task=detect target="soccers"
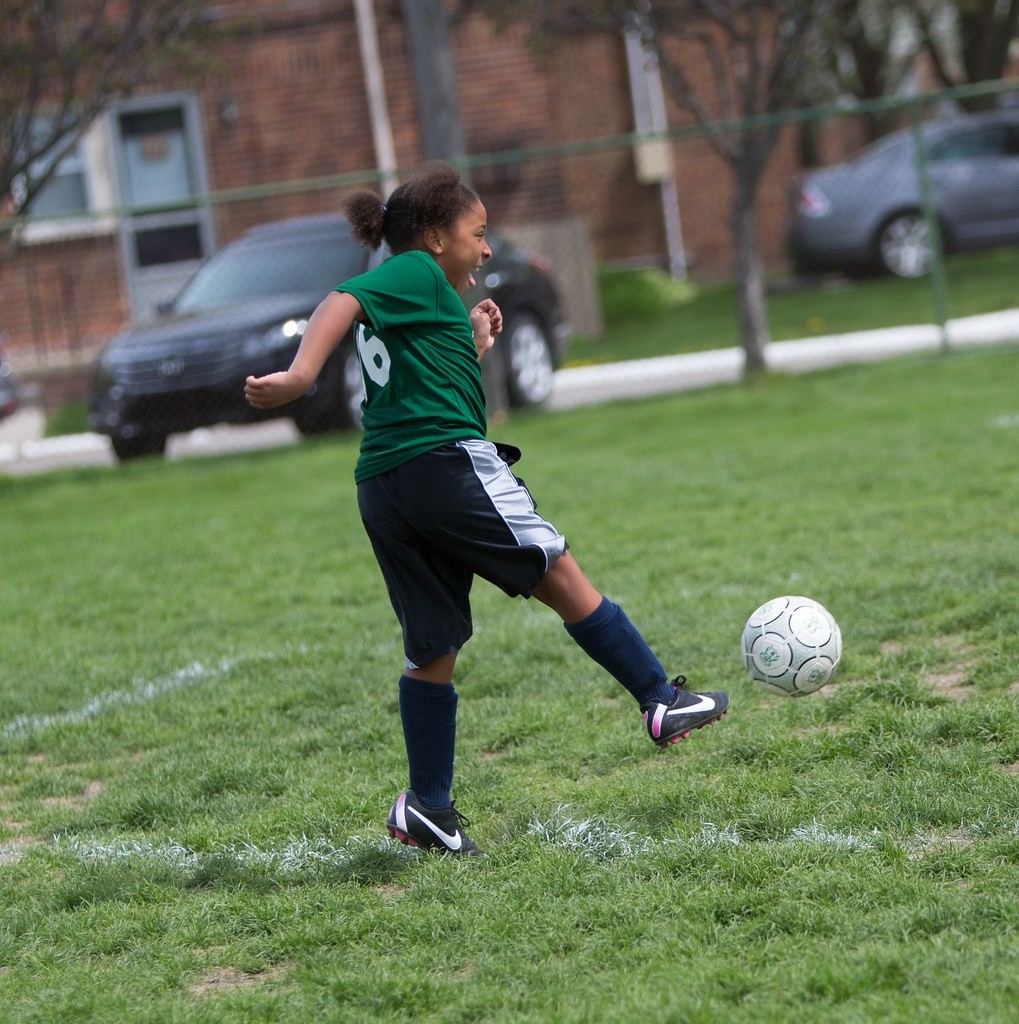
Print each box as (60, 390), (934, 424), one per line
(740, 595), (844, 698)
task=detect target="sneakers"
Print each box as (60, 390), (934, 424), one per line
(388, 788), (483, 858)
(646, 675), (730, 748)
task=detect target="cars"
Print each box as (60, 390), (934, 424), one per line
(87, 208), (571, 466)
(781, 110), (1017, 282)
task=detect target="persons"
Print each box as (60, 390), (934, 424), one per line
(243, 168), (729, 855)
